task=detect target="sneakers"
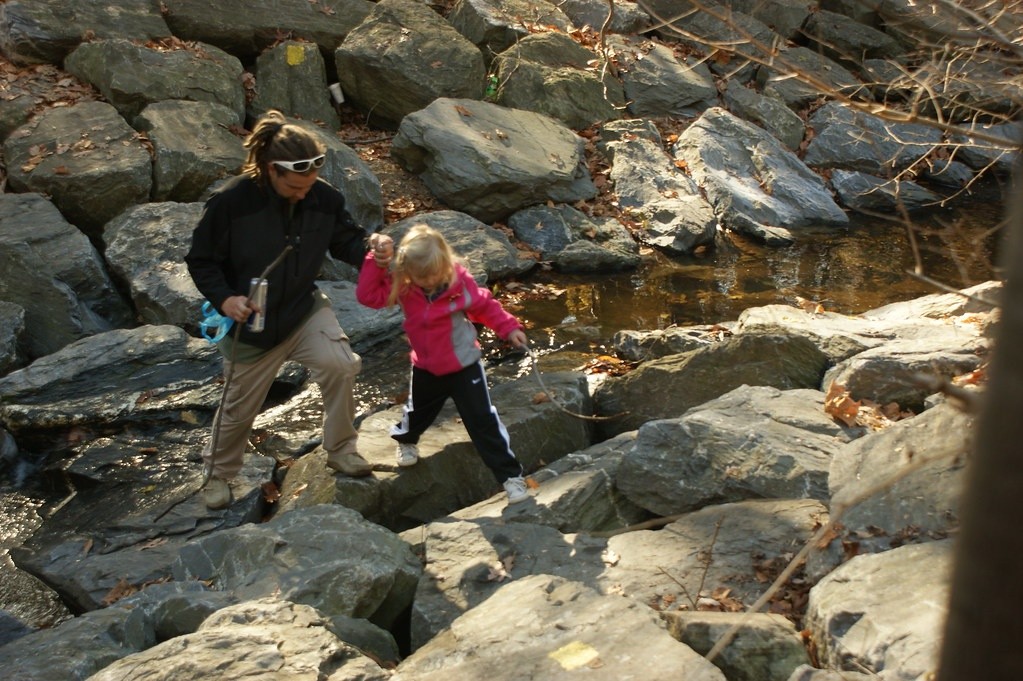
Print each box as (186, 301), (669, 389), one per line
(503, 477), (528, 504)
(396, 442), (419, 466)
(202, 466), (230, 508)
(327, 452), (373, 476)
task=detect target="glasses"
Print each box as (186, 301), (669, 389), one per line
(268, 154), (326, 172)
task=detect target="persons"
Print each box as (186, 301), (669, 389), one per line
(183, 109), (395, 510)
(356, 223), (530, 505)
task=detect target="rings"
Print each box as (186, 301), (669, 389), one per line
(388, 256), (392, 262)
